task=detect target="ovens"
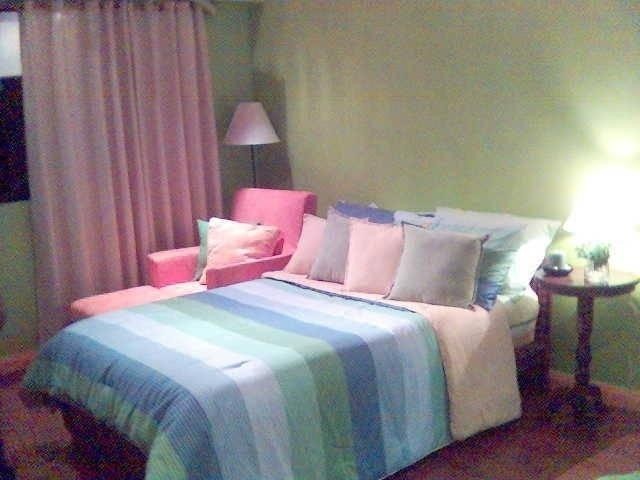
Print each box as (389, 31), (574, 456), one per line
(70, 189), (317, 320)
(18, 281), (550, 480)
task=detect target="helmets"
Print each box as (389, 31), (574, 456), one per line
(542, 262), (574, 275)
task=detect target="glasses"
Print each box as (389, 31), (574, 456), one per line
(224, 103), (281, 189)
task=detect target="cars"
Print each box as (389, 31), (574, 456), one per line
(550, 254), (563, 266)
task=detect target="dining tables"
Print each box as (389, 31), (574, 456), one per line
(576, 243), (611, 286)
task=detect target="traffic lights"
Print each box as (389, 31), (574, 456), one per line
(200, 217), (281, 284)
(192, 219), (208, 281)
(286, 202), (562, 311)
